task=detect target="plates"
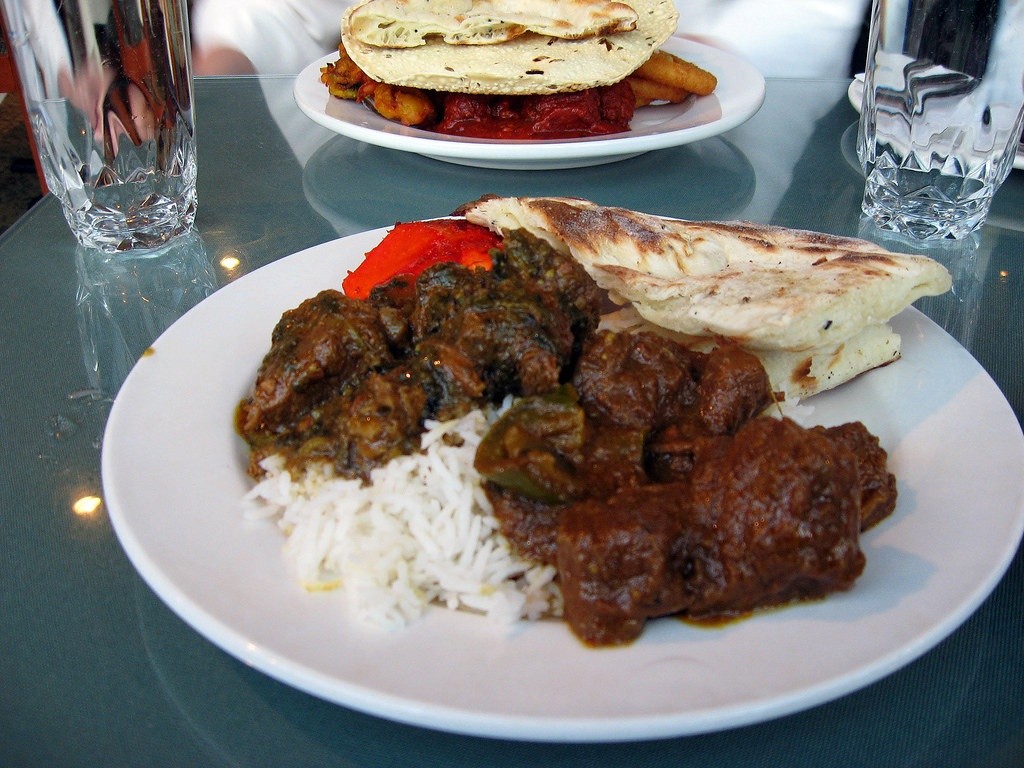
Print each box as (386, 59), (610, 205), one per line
(301, 135), (756, 235)
(99, 221), (1024, 743)
(848, 79), (1024, 170)
(293, 38), (767, 170)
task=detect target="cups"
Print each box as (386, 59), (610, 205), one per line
(0, 0), (198, 251)
(77, 238), (217, 392)
(848, 212), (986, 350)
(857, 0), (1024, 244)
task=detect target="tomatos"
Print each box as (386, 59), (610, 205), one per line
(342, 220), (504, 296)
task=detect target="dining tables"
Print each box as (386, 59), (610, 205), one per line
(0, 77), (1024, 768)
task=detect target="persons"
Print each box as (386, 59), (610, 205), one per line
(190, 0), (870, 79)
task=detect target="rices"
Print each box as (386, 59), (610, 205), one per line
(245, 396), (564, 628)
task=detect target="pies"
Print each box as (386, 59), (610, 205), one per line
(340, 0), (679, 96)
(465, 196), (952, 399)
